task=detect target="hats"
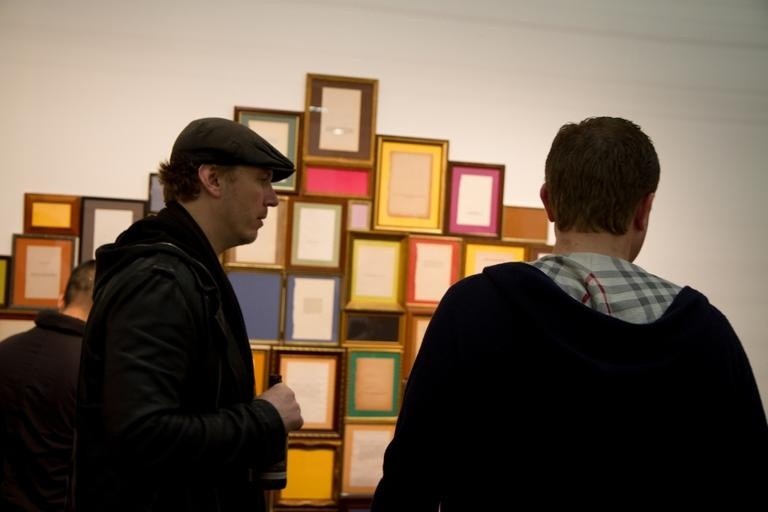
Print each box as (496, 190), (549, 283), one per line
(171, 118), (295, 183)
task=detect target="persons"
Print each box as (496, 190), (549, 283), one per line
(365, 114), (768, 511)
(1, 260), (100, 510)
(70, 118), (303, 511)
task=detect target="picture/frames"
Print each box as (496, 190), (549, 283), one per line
(2, 172), (166, 338)
(408, 233), (556, 310)
(340, 227), (411, 314)
(246, 345), (341, 512)
(444, 159), (506, 239)
(220, 198), (340, 347)
(301, 73), (379, 171)
(340, 311), (433, 425)
(370, 134), (449, 234)
(232, 105), (305, 196)
(286, 195), (349, 277)
